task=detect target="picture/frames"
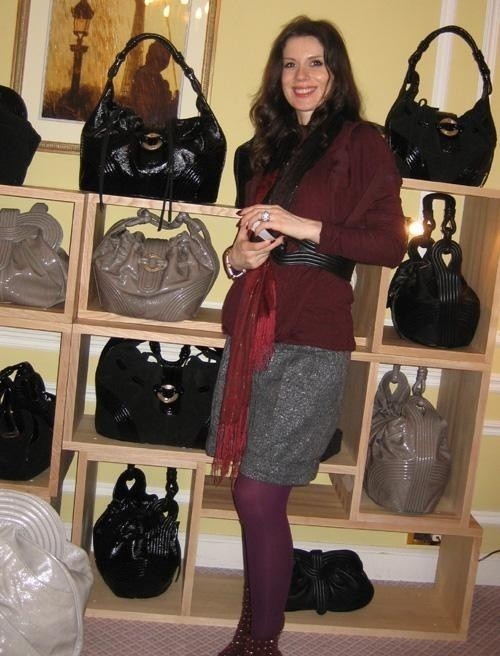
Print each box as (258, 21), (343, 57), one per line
(11, 0), (221, 157)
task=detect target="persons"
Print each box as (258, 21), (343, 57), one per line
(131, 40), (171, 126)
(205, 17), (408, 656)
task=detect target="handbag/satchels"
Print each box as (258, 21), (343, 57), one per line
(95, 336), (223, 449)
(92, 465), (181, 598)
(0, 361), (56, 481)
(285, 548), (374, 613)
(0, 85), (41, 185)
(0, 203), (69, 308)
(79, 33), (226, 204)
(91, 208), (220, 322)
(362, 364), (452, 514)
(385, 24), (496, 189)
(0, 489), (95, 656)
(386, 192), (481, 350)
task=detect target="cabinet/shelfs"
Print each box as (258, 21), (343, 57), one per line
(0, 184), (85, 323)
(190, 516), (483, 642)
(62, 324), (228, 463)
(371, 179), (499, 364)
(0, 317), (73, 497)
(78, 193), (243, 333)
(348, 355), (490, 527)
(350, 262), (382, 354)
(71, 451), (200, 623)
(202, 459), (355, 520)
(318, 352), (377, 475)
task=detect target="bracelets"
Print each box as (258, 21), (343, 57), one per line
(224, 249), (247, 279)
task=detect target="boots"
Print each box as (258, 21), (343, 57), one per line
(218, 582), (285, 655)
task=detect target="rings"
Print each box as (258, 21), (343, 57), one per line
(262, 210), (270, 221)
